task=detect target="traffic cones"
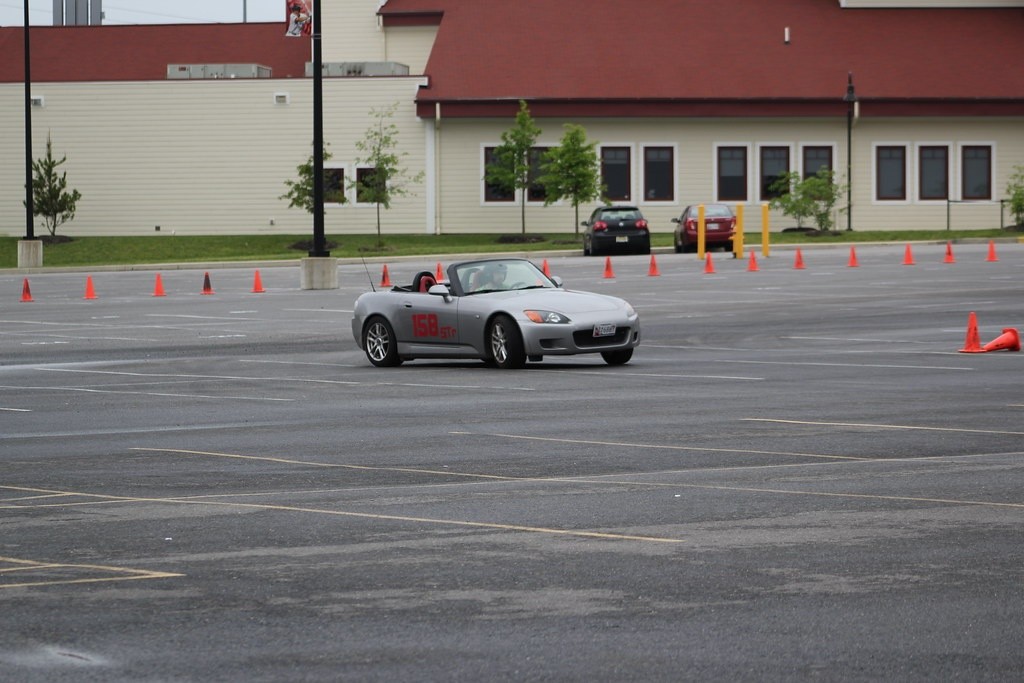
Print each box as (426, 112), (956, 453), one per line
(984, 240), (1001, 262)
(537, 258), (551, 285)
(646, 254), (661, 277)
(901, 243), (916, 265)
(704, 253), (716, 274)
(200, 271), (216, 295)
(602, 256), (616, 279)
(792, 248), (806, 270)
(19, 278), (36, 303)
(956, 311), (987, 352)
(848, 243), (862, 267)
(942, 240), (958, 262)
(251, 270), (266, 294)
(378, 264), (394, 287)
(747, 250), (760, 271)
(151, 271), (167, 297)
(982, 327), (1022, 350)
(81, 275), (99, 299)
(435, 261), (443, 281)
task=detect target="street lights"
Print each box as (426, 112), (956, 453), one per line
(840, 70), (857, 234)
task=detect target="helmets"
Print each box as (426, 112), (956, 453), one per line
(483, 263), (508, 283)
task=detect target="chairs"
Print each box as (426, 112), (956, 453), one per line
(461, 267), (481, 293)
(411, 271), (437, 294)
(624, 214), (633, 220)
(602, 215), (613, 221)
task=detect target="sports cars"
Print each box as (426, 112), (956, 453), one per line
(349, 255), (643, 370)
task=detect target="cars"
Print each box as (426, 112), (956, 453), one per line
(671, 203), (737, 253)
(580, 204), (652, 257)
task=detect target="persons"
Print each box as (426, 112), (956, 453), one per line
(474, 264), (510, 293)
(285, 2), (312, 36)
